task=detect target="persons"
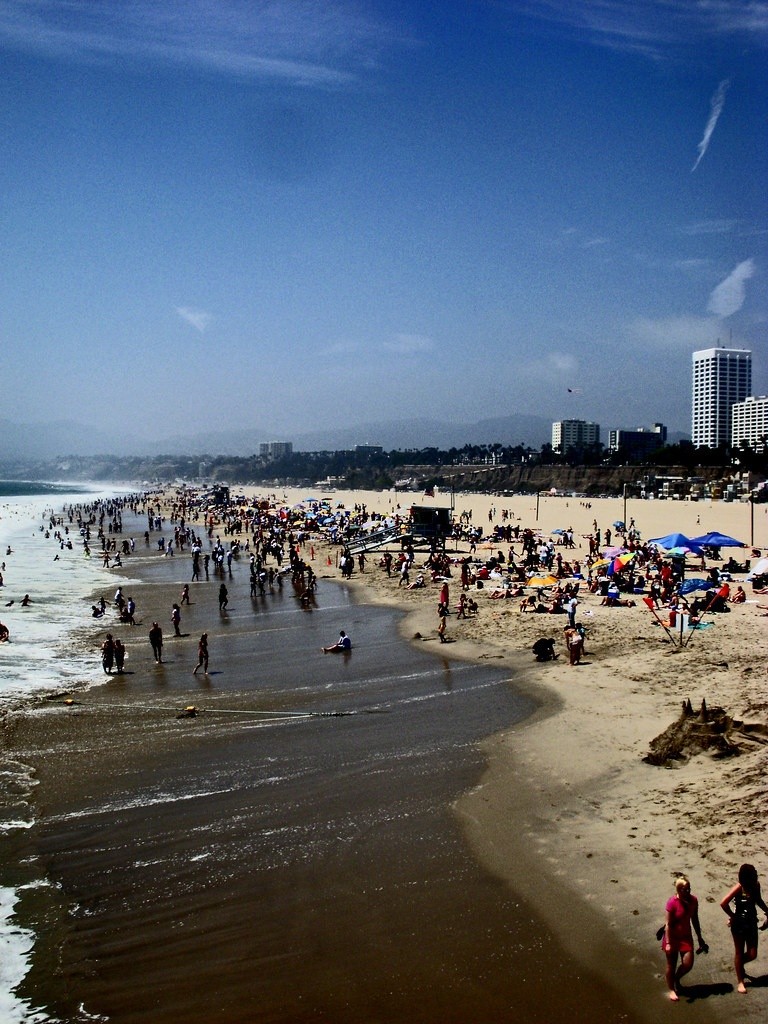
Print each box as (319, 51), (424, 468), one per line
(719, 864), (768, 993)
(0, 478), (767, 676)
(661, 875), (705, 1002)
